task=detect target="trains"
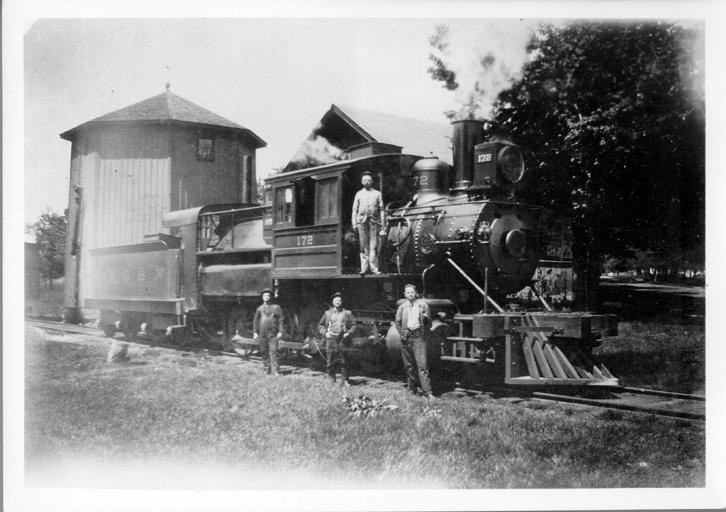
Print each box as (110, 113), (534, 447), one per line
(83, 118), (623, 387)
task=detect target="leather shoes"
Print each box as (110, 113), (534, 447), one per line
(425, 393), (438, 400)
(271, 367), (281, 378)
(342, 378), (352, 388)
(370, 266), (382, 275)
(357, 264), (369, 275)
(326, 379), (337, 383)
(262, 368), (271, 376)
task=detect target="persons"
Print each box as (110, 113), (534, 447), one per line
(349, 169), (387, 274)
(394, 284), (436, 401)
(317, 291), (356, 388)
(252, 287), (283, 377)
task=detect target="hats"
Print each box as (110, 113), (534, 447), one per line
(330, 291), (343, 301)
(360, 170), (375, 177)
(260, 288), (275, 298)
